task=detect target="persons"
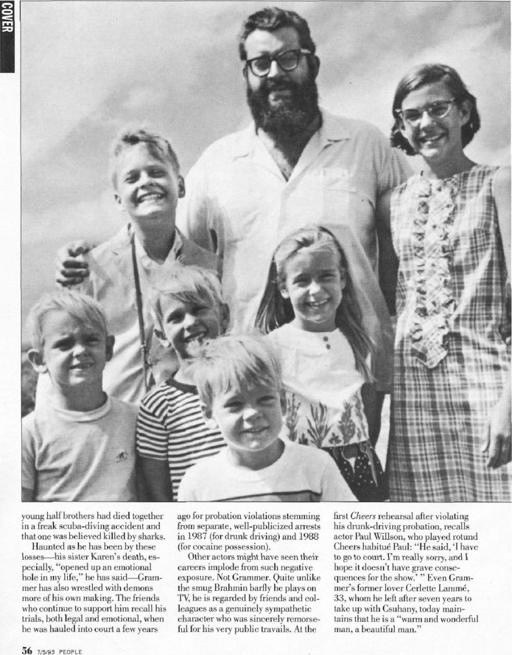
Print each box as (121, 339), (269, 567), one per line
(175, 332), (360, 503)
(54, 6), (414, 451)
(136, 262), (233, 502)
(54, 124), (222, 409)
(20, 288), (141, 503)
(373, 59), (510, 502)
(242, 223), (391, 502)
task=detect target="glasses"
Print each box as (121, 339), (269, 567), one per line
(396, 97), (458, 129)
(246, 48), (312, 78)
